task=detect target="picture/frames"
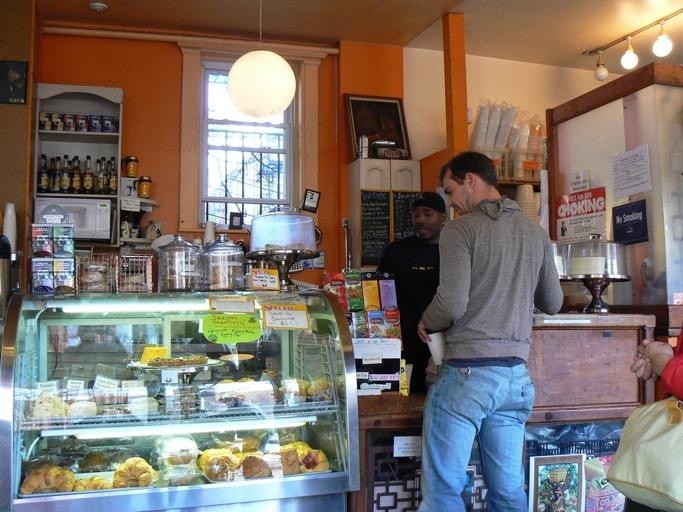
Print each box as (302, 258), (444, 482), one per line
(228, 210), (244, 230)
(340, 91), (413, 160)
(527, 455), (586, 512)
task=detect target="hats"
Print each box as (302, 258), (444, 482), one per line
(413, 191), (445, 213)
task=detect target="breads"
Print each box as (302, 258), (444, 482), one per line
(21, 378), (329, 428)
(20, 428), (329, 492)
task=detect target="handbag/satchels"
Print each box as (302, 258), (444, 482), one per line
(605, 396), (681, 512)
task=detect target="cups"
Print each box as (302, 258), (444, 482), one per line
(516, 183), (542, 224)
(508, 119), (545, 180)
(120, 155), (139, 178)
(424, 332), (447, 368)
(133, 176), (153, 198)
(467, 102), (516, 179)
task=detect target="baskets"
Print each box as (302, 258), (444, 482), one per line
(113, 254), (153, 294)
(74, 253), (113, 295)
(525, 438), (619, 482)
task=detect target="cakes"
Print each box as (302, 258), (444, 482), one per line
(566, 257), (604, 274)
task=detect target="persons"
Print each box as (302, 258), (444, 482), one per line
(560, 220), (567, 235)
(416, 151), (566, 510)
(375, 192), (446, 393)
(604, 325), (683, 512)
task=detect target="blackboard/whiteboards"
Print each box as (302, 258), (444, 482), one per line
(392, 190), (422, 242)
(359, 189), (391, 268)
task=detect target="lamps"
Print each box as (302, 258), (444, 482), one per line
(217, 346), (256, 361)
(581, 9), (682, 81)
(223, 0), (296, 121)
(89, 1), (109, 12)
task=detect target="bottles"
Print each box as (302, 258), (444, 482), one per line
(356, 135), (368, 159)
(201, 233), (245, 291)
(155, 230), (201, 293)
(36, 152), (117, 195)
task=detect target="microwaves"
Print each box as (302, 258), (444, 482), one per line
(34, 196), (111, 241)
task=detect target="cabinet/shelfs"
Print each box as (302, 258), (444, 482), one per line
(345, 312), (660, 512)
(13, 313), (345, 498)
(30, 82), (124, 250)
(116, 196), (161, 247)
(347, 160), (421, 271)
(474, 147), (549, 189)
